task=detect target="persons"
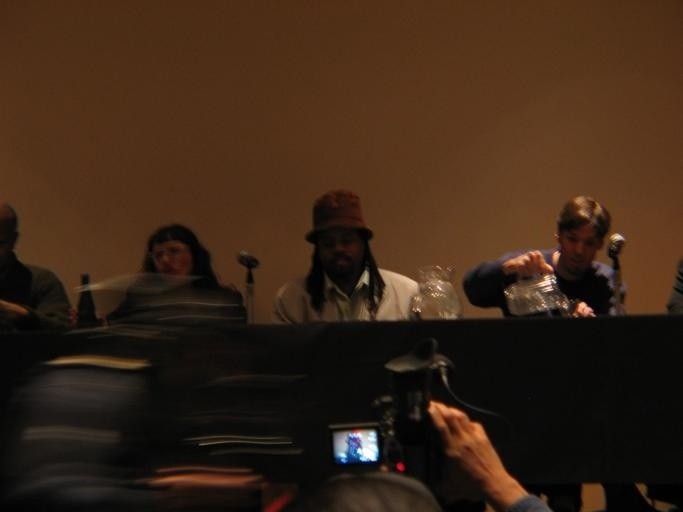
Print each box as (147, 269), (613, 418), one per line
(457, 195), (629, 316)
(0, 196), (74, 335)
(89, 221), (248, 322)
(277, 391), (555, 511)
(272, 185), (425, 326)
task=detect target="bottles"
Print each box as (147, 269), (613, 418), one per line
(76, 273), (97, 329)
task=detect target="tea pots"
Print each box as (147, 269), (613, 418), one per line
(409, 264), (463, 320)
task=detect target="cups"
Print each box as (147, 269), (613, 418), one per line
(505, 269), (564, 315)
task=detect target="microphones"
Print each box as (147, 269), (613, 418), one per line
(238, 250), (260, 270)
(610, 233), (626, 253)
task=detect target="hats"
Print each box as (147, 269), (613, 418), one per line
(302, 188), (373, 243)
